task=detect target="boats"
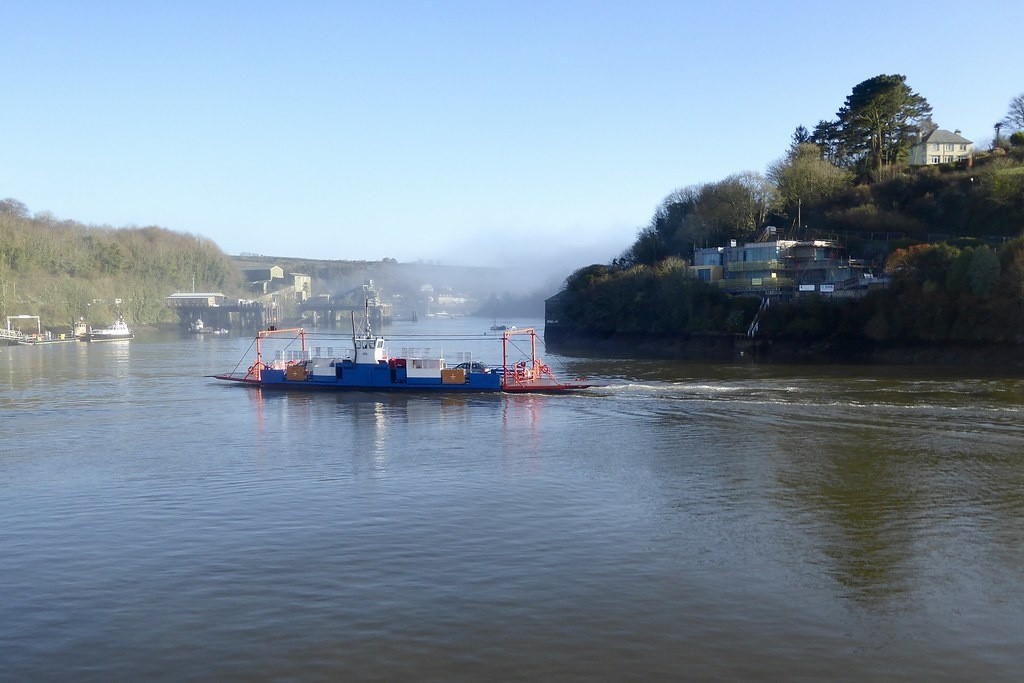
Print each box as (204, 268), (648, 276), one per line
(488, 316), (516, 333)
(70, 312), (134, 340)
(206, 296), (608, 392)
